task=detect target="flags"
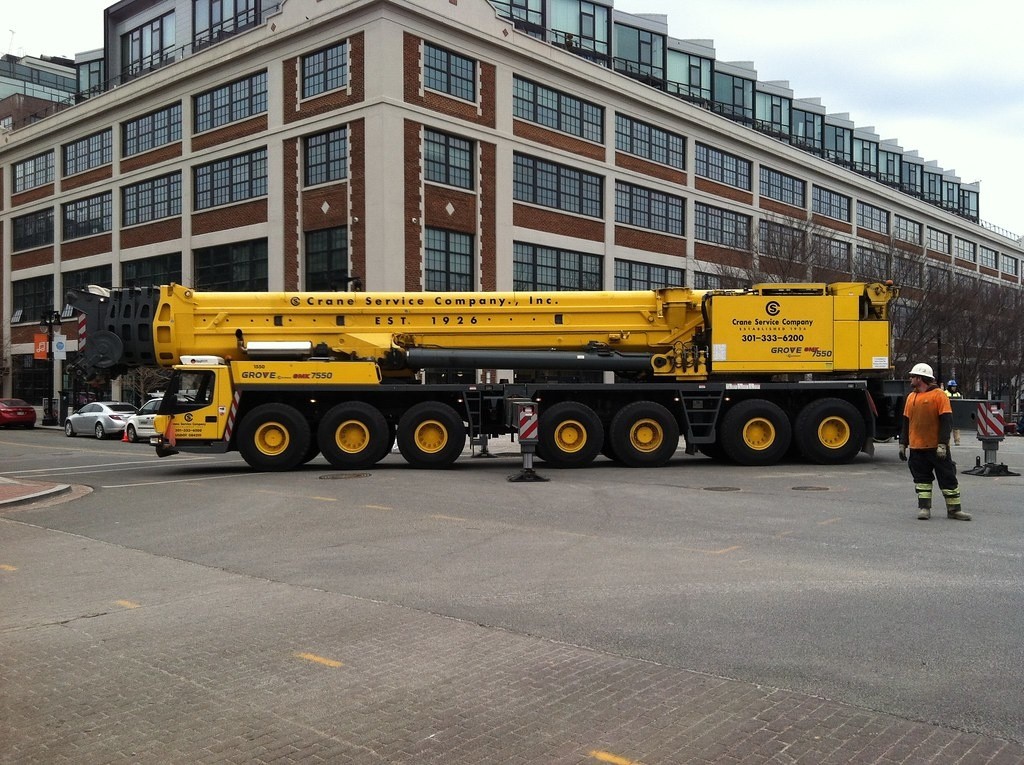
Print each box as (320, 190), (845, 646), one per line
(166, 421), (175, 446)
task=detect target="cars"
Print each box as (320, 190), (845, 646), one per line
(65, 402), (139, 440)
(125, 398), (164, 443)
(0, 398), (36, 430)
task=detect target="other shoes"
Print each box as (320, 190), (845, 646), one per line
(917, 508), (930, 519)
(955, 440), (960, 446)
(947, 510), (972, 520)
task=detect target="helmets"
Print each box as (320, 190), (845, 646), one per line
(908, 363), (935, 380)
(948, 380), (957, 386)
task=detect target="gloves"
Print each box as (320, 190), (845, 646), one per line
(936, 444), (948, 463)
(898, 444), (908, 461)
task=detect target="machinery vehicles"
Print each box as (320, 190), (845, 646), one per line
(65, 280), (904, 483)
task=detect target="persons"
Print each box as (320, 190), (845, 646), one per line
(899, 363), (972, 521)
(943, 380), (963, 445)
